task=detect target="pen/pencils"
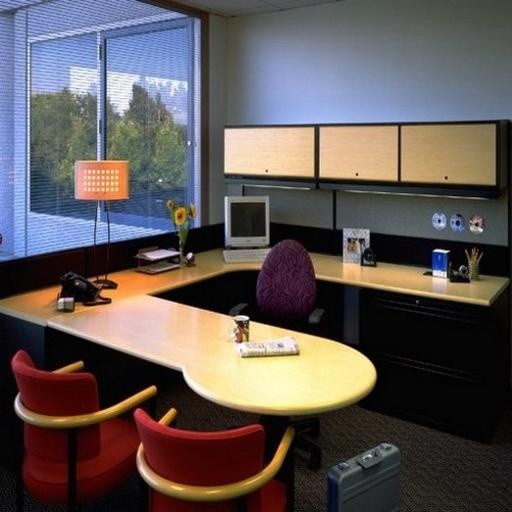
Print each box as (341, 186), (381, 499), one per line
(465, 247), (483, 280)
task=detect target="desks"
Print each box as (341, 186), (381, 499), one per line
(0, 234), (512, 493)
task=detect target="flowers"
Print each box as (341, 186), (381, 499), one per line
(165, 199), (198, 260)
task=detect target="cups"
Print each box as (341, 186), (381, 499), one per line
(467, 260), (479, 280)
(233, 315), (249, 344)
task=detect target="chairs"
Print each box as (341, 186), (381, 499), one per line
(10, 349), (158, 512)
(233, 236), (328, 473)
(133, 405), (295, 512)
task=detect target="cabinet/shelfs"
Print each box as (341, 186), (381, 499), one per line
(219, 112), (512, 198)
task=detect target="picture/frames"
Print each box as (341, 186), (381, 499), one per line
(342, 227), (370, 264)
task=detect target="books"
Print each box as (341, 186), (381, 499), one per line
(235, 336), (299, 357)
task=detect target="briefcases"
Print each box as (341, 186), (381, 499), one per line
(327, 443), (401, 512)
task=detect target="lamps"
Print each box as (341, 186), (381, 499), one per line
(70, 159), (130, 288)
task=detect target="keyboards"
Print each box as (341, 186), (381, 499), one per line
(223, 249), (272, 264)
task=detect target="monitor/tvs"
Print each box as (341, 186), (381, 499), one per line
(225, 196), (271, 249)
